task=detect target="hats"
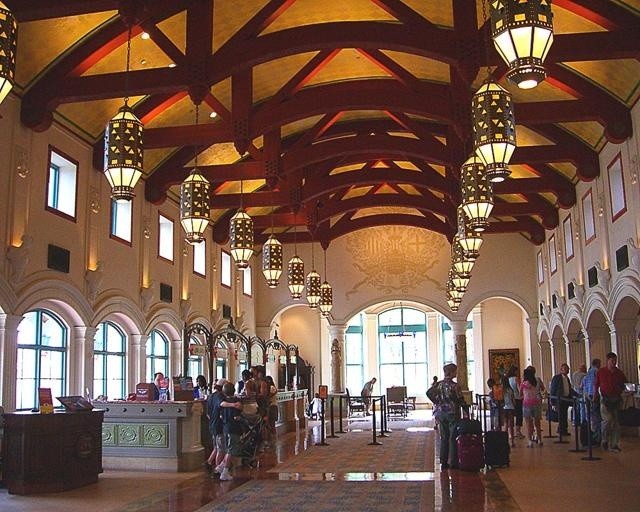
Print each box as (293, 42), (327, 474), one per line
(216, 379), (234, 392)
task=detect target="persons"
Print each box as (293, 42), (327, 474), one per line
(431, 374), (439, 385)
(305, 391), (324, 422)
(145, 364), (278, 479)
(488, 349), (630, 456)
(361, 377), (375, 412)
(426, 364), (468, 469)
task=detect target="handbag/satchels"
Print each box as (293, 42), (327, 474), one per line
(618, 407), (640, 426)
(457, 419), (481, 434)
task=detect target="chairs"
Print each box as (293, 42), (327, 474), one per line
(345, 387), (366, 417)
(387, 384), (408, 420)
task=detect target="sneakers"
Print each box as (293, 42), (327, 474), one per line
(201, 461), (233, 481)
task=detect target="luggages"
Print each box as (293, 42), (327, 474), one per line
(456, 434), (485, 470)
(484, 432), (509, 469)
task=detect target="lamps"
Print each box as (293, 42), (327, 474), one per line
(485, 1), (557, 90)
(263, 173), (284, 291)
(178, 105), (212, 246)
(460, 149), (495, 234)
(289, 203), (305, 302)
(319, 245), (334, 314)
(470, 57), (518, 183)
(0, 2), (18, 104)
(229, 153), (254, 270)
(105, 20), (144, 205)
(444, 202), (485, 312)
(307, 224), (323, 308)
